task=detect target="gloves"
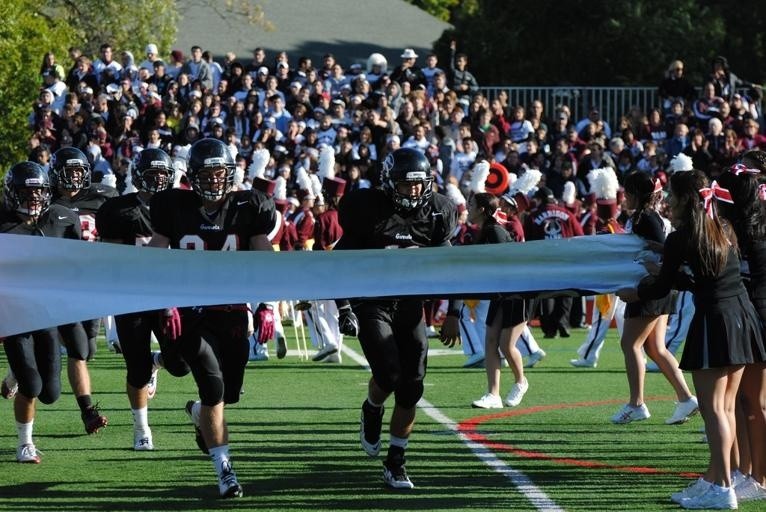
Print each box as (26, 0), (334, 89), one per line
(161, 307), (183, 341)
(252, 302), (275, 344)
(338, 309), (361, 337)
(433, 297), (451, 322)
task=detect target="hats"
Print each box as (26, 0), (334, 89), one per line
(400, 48), (419, 59)
(258, 65), (269, 76)
(171, 49), (183, 62)
(275, 200), (290, 215)
(251, 176), (276, 197)
(594, 197), (617, 217)
(276, 61), (289, 71)
(297, 188), (315, 200)
(616, 188), (625, 203)
(501, 192), (529, 215)
(566, 192), (597, 217)
(313, 195), (324, 206)
(531, 187), (556, 204)
(456, 205), (467, 214)
(322, 177), (347, 197)
(146, 44), (159, 55)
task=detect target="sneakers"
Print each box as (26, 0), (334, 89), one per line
(460, 350), (485, 368)
(274, 334), (287, 358)
(381, 457), (415, 490)
(185, 400), (213, 456)
(311, 343), (338, 361)
(358, 406), (384, 456)
(216, 460), (244, 500)
(248, 347), (269, 360)
(499, 358), (510, 368)
(557, 322), (571, 338)
(504, 375), (528, 407)
(82, 408), (107, 435)
(571, 357), (596, 369)
(15, 442), (40, 464)
(471, 393), (504, 410)
(326, 351), (344, 365)
(1, 365), (22, 399)
(524, 348), (546, 367)
(147, 350), (161, 399)
(134, 426), (153, 452)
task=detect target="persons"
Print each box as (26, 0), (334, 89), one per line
(2, 40), (766, 512)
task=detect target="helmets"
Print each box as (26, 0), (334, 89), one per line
(379, 148), (436, 215)
(48, 146), (93, 192)
(130, 147), (176, 194)
(184, 137), (236, 202)
(4, 161), (51, 221)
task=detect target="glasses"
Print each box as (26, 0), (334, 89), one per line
(672, 67), (684, 72)
